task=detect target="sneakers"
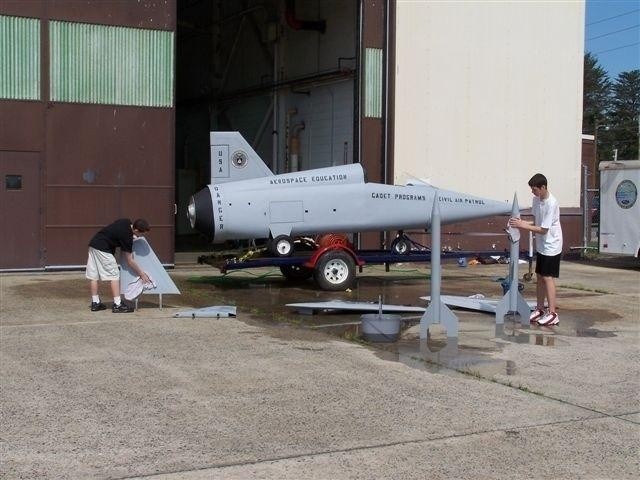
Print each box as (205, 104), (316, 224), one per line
(92, 302), (107, 311)
(113, 303), (134, 313)
(530, 309), (559, 325)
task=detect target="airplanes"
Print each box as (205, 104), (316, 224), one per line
(188, 131), (531, 258)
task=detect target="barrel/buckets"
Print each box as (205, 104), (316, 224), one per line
(360, 314), (403, 344)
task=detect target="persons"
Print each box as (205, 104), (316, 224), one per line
(84, 217), (151, 314)
(508, 171), (564, 326)
